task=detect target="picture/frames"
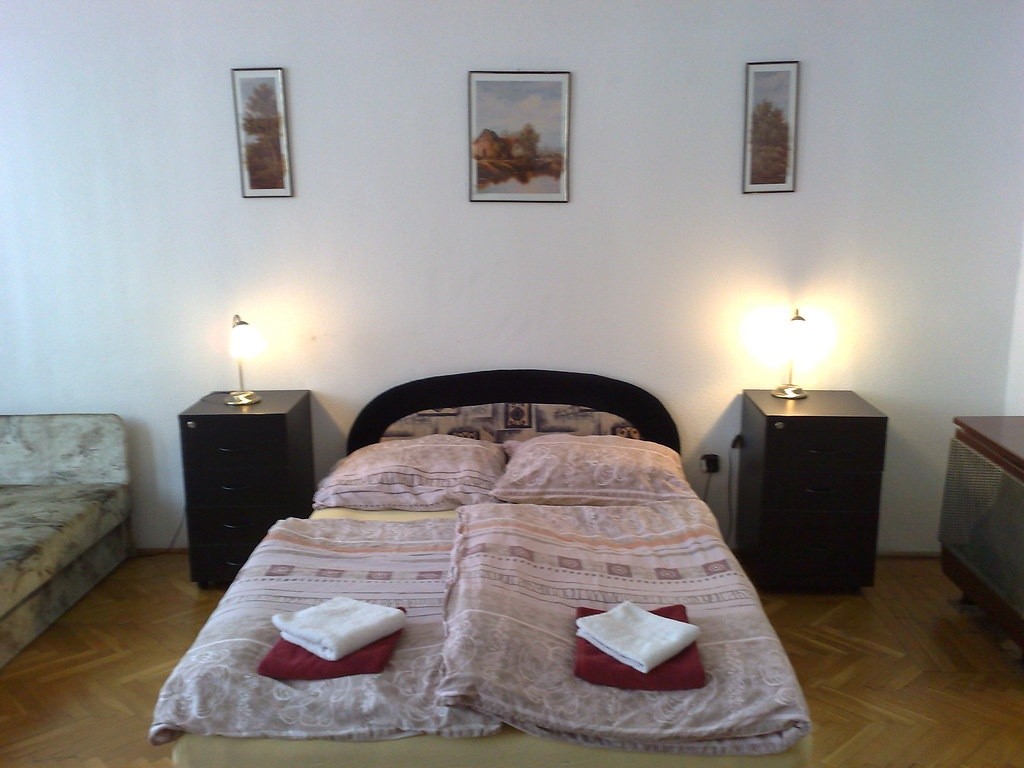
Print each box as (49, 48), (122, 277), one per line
(231, 67), (293, 199)
(741, 61), (800, 194)
(467, 70), (571, 202)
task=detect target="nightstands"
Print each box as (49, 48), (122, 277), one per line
(735, 390), (889, 591)
(178, 390), (318, 590)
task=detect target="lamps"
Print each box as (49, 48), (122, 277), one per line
(223, 314), (263, 407)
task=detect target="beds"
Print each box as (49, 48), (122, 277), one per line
(151, 371), (812, 768)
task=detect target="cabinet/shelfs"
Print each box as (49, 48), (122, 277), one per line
(936, 415), (1024, 653)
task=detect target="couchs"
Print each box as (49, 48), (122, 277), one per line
(0, 413), (134, 670)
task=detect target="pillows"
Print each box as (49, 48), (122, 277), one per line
(489, 432), (699, 506)
(313, 434), (507, 514)
